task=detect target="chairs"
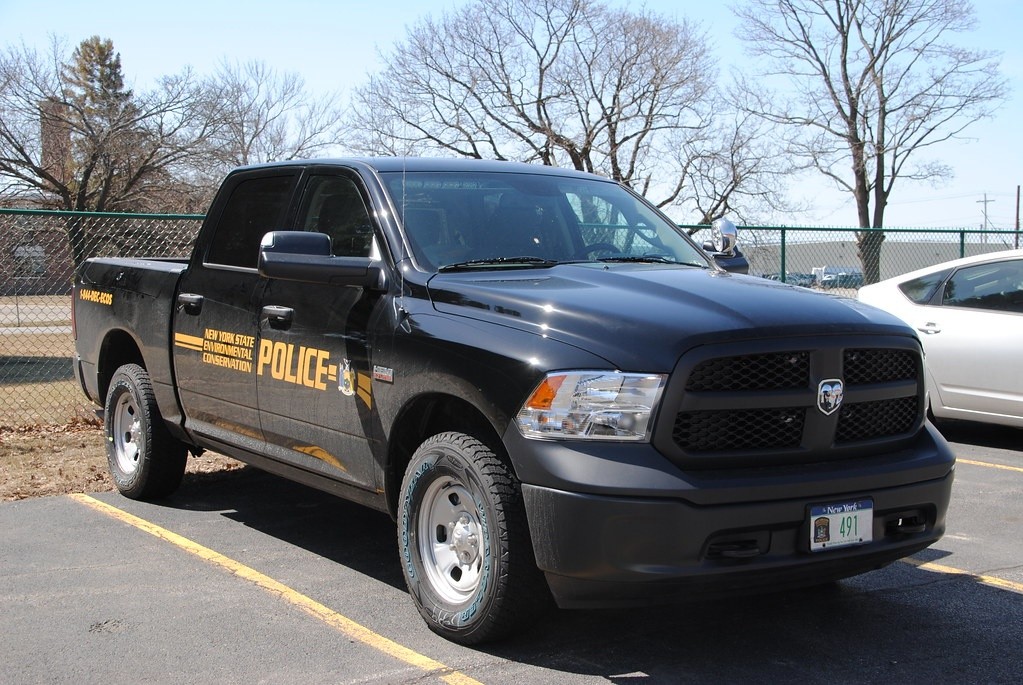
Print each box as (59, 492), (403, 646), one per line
(318, 194), (373, 259)
(401, 206), (441, 258)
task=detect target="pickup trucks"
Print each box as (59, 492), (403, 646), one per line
(73, 158), (957, 646)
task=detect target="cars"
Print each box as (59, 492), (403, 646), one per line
(766, 273), (864, 288)
(857, 248), (1023, 430)
